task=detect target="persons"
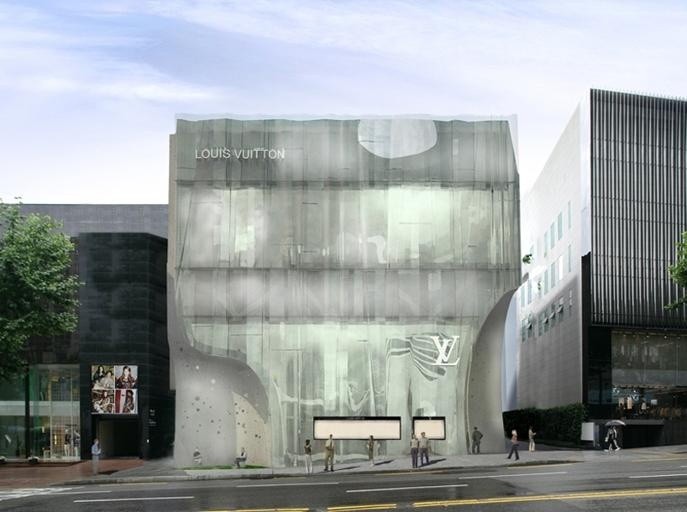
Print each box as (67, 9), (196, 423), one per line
(117, 365), (137, 388)
(507, 429), (519, 461)
(528, 426), (537, 452)
(618, 398), (683, 421)
(93, 370), (113, 389)
(90, 438), (102, 476)
(603, 426), (618, 452)
(123, 390), (135, 413)
(324, 433), (334, 472)
(232, 447), (247, 469)
(471, 426), (483, 454)
(94, 364), (105, 381)
(410, 434), (419, 468)
(419, 431), (432, 465)
(192, 447), (203, 466)
(94, 391), (110, 414)
(367, 435), (375, 467)
(303, 438), (314, 474)
(610, 427), (621, 451)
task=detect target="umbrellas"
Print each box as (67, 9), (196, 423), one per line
(604, 419), (626, 426)
